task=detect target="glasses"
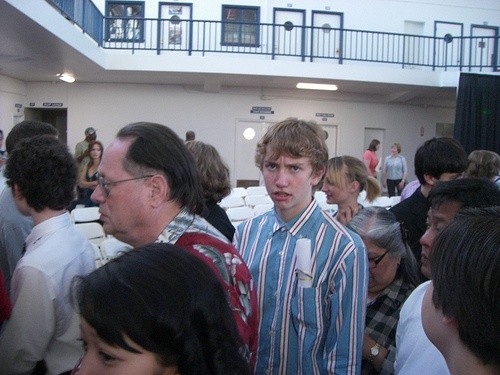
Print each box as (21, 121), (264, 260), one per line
(368, 249), (390, 268)
(94, 169), (156, 198)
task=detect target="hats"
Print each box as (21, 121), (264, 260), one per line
(85, 127), (96, 136)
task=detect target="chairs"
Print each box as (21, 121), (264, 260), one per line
(70, 187), (401, 268)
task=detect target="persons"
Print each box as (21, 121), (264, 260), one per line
(322, 156), (379, 225)
(0, 120), (59, 324)
(70, 245), (254, 375)
(80, 142), (103, 207)
(393, 178), (500, 375)
(187, 142), (236, 243)
(387, 137), (470, 266)
(383, 143), (407, 198)
(0, 135), (98, 375)
(346, 206), (420, 375)
(362, 139), (381, 178)
(464, 150), (500, 190)
(90, 122), (260, 370)
(74, 127), (97, 200)
(233, 119), (369, 375)
(421, 206), (500, 375)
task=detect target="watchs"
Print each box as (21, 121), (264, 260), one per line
(368, 342), (382, 363)
